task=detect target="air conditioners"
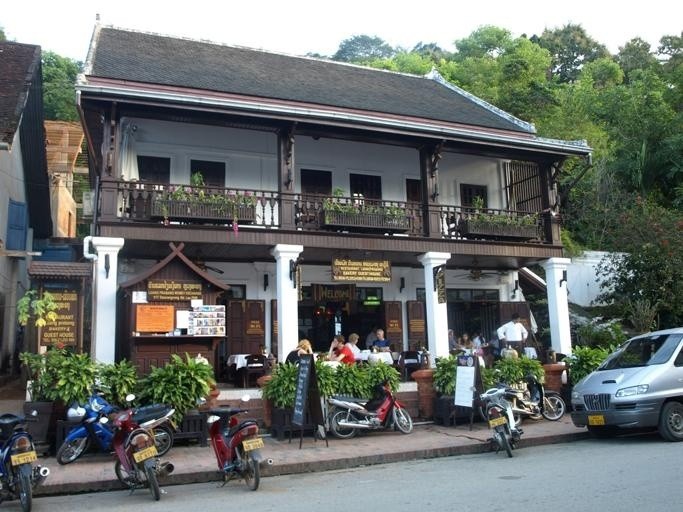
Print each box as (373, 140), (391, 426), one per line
(82, 191), (95, 216)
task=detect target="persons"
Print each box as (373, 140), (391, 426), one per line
(496, 312), (529, 357)
(472, 330), (485, 348)
(365, 326), (377, 350)
(448, 329), (462, 351)
(326, 335), (355, 369)
(371, 329), (391, 353)
(457, 333), (475, 350)
(344, 333), (361, 354)
(285, 340), (318, 368)
(487, 335), (500, 356)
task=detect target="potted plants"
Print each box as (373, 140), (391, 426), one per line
(432, 355), (465, 427)
(17, 350), (67, 446)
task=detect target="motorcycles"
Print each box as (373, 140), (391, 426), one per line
(0, 409), (49, 511)
(470, 382), (522, 457)
(99, 394), (174, 500)
(55, 387), (177, 465)
(197, 392), (273, 492)
(492, 364), (565, 426)
(323, 378), (414, 439)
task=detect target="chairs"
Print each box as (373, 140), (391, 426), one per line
(400, 351), (423, 381)
(245, 354), (267, 388)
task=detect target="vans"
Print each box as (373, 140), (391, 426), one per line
(570, 328), (682, 442)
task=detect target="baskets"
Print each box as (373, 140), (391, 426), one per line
(206, 422), (213, 432)
(66, 413), (86, 424)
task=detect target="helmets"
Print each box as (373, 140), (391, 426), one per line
(67, 407), (81, 421)
(206, 415), (220, 427)
